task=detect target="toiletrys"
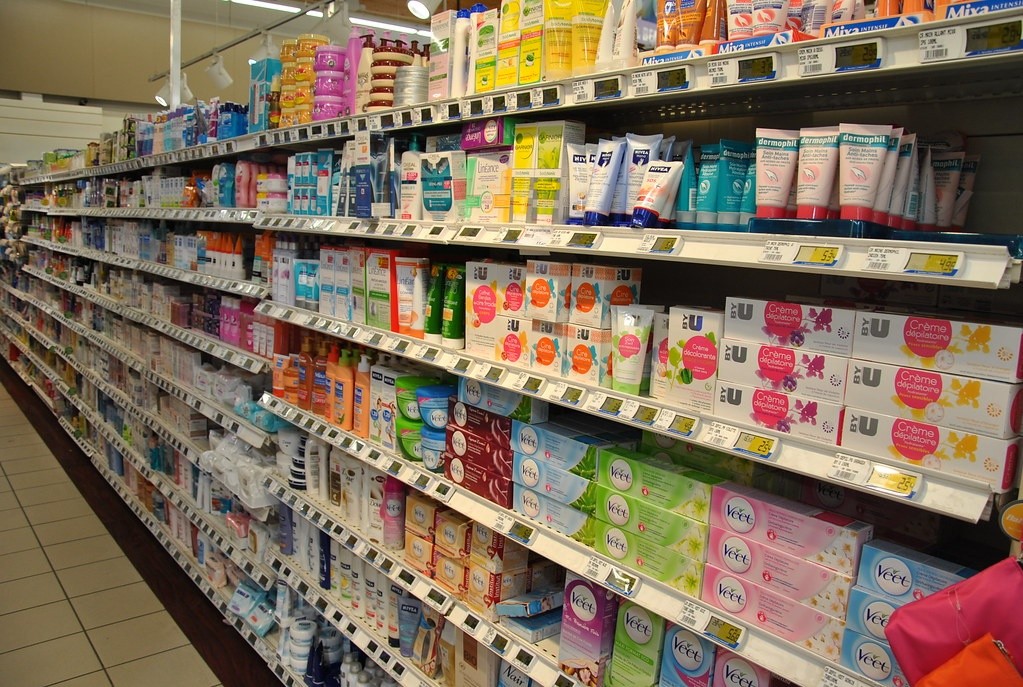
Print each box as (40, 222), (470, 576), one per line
(0, 1), (1023, 685)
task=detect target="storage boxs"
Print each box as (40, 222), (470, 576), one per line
(105, 222), (142, 259)
(159, 336), (201, 386)
(318, 242), (1023, 687)
(476, 0), (544, 96)
(174, 236), (197, 270)
(152, 283), (191, 328)
(428, 10), (458, 102)
(248, 58), (283, 134)
(164, 396), (207, 441)
(161, 177), (192, 209)
(420, 121), (583, 226)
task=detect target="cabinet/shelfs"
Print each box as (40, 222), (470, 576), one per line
(0, 0), (1023, 687)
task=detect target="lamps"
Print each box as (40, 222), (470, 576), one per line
(248, 33), (279, 65)
(156, 70), (193, 106)
(206, 56), (233, 91)
(407, 0), (447, 20)
